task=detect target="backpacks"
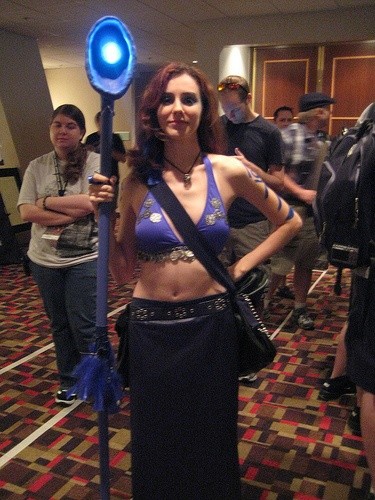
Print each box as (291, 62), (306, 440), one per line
(312, 127), (375, 295)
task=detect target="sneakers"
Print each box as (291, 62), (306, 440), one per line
(54, 388), (77, 405)
(295, 313), (315, 329)
(318, 374), (356, 401)
(348, 405), (361, 431)
(262, 304), (271, 320)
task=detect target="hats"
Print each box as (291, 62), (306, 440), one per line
(298, 93), (336, 112)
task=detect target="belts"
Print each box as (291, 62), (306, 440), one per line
(130, 294), (233, 320)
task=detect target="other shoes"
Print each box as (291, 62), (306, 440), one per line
(238, 373), (258, 383)
(276, 286), (295, 299)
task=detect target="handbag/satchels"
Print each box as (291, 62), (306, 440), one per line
(231, 291), (277, 377)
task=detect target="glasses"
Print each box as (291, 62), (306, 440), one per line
(217, 82), (248, 92)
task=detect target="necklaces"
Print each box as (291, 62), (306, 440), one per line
(164, 148), (201, 190)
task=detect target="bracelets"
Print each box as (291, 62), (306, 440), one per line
(43, 195), (52, 211)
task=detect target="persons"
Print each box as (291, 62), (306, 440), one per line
(263, 92), (336, 329)
(319, 103), (375, 500)
(212, 76), (284, 381)
(17, 104), (100, 406)
(85, 111), (127, 231)
(88, 62), (302, 500)
